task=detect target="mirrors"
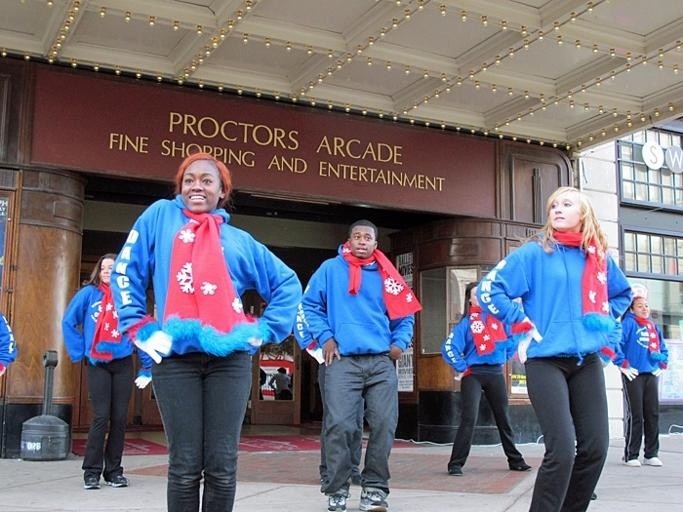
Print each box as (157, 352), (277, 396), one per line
(257, 302), (295, 402)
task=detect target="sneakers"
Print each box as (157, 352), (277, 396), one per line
(351, 474), (365, 485)
(447, 462), (465, 476)
(82, 469), (101, 490)
(641, 456), (663, 466)
(359, 487), (389, 512)
(102, 472), (130, 490)
(621, 457), (641, 467)
(509, 460), (533, 471)
(327, 486), (347, 512)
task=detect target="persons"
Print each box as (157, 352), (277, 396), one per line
(61, 251), (152, 490)
(475, 185), (637, 512)
(0, 309), (18, 376)
(108, 151), (302, 512)
(292, 239), (364, 485)
(259, 363), (293, 400)
(611, 295), (669, 468)
(590, 320), (622, 500)
(441, 280), (531, 478)
(292, 217), (417, 512)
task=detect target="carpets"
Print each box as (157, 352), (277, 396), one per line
(69, 435), (169, 457)
(119, 451), (541, 492)
(235, 432), (421, 452)
